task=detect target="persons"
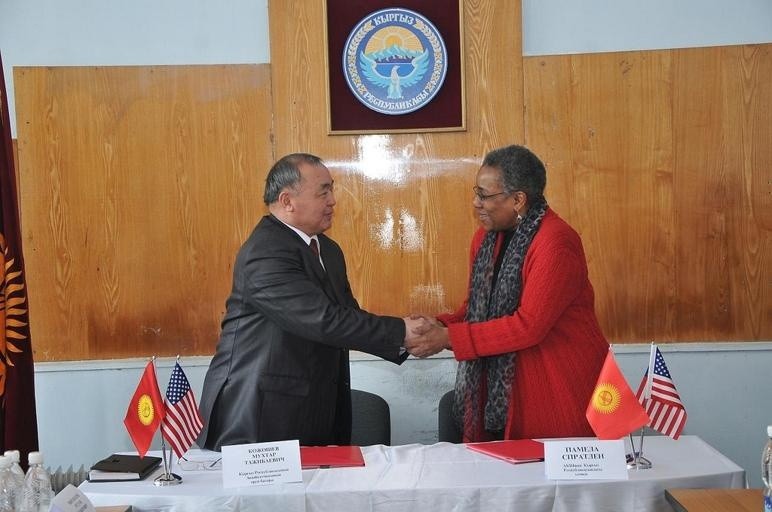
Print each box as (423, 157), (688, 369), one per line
(192, 152), (435, 453)
(403, 144), (612, 440)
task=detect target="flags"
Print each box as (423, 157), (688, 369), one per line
(160, 361), (205, 460)
(0, 52), (41, 457)
(585, 350), (650, 440)
(634, 346), (687, 441)
(123, 361), (167, 459)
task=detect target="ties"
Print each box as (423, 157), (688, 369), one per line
(310, 238), (319, 269)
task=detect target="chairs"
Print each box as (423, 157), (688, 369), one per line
(438, 389), (461, 444)
(351, 388), (390, 445)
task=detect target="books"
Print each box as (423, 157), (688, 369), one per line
(458, 438), (545, 465)
(295, 444), (367, 472)
(87, 452), (163, 484)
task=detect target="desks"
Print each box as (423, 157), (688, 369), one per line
(662, 486), (772, 512)
(75, 433), (749, 511)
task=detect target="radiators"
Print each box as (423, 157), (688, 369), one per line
(43, 463), (96, 493)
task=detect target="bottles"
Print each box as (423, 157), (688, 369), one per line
(0, 449), (53, 511)
(759, 425), (771, 511)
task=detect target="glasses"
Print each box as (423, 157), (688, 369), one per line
(472, 186), (504, 201)
